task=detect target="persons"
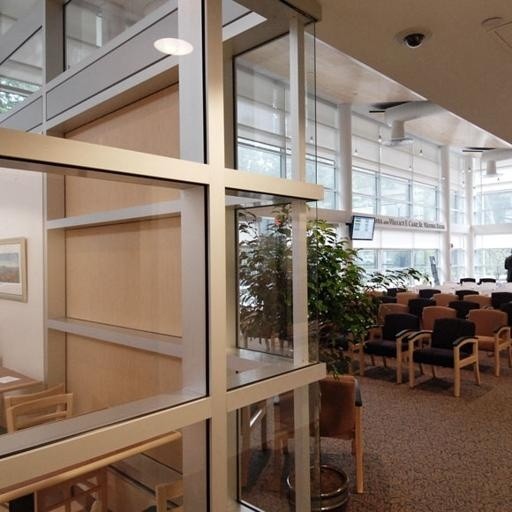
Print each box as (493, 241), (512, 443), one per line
(503, 247), (512, 283)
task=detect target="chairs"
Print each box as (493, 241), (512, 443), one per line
(237, 357), (270, 488)
(272, 373), (364, 511)
(146, 479), (183, 512)
(0, 383), (65, 433)
(4, 392), (108, 512)
(355, 277), (512, 398)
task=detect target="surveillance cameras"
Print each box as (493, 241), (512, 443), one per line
(395, 27), (431, 49)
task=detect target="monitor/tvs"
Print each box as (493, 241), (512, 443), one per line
(350, 216), (376, 240)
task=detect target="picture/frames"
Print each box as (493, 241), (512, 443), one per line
(0, 237), (28, 304)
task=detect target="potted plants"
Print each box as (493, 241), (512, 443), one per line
(238, 200), (432, 512)
